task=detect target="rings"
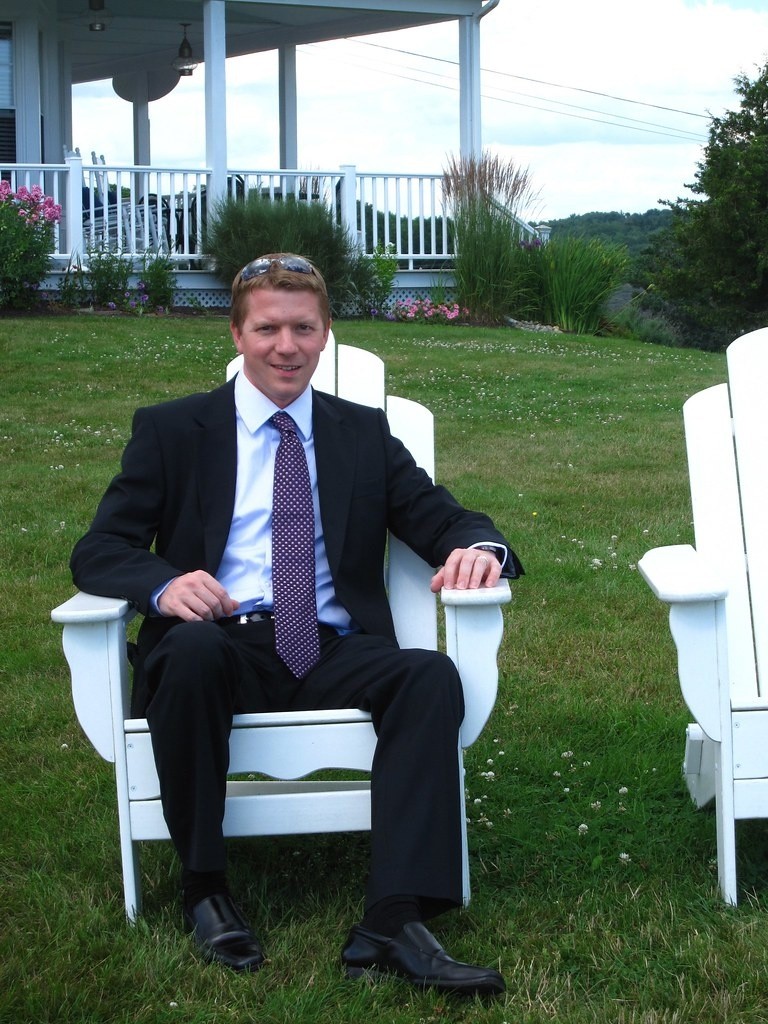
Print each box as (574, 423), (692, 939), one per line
(477, 556), (488, 566)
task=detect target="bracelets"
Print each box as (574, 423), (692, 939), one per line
(480, 546), (496, 553)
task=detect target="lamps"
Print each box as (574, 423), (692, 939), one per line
(171, 24), (198, 76)
(80, 0), (114, 31)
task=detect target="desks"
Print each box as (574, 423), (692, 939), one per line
(261, 192), (319, 203)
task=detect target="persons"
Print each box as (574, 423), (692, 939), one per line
(69, 252), (526, 998)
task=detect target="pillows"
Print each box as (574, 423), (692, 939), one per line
(83, 187), (103, 218)
(108, 191), (117, 214)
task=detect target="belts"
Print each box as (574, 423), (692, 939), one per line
(217, 610), (276, 630)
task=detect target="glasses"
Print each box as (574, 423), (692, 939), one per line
(237, 256), (318, 287)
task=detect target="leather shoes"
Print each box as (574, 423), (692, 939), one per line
(341, 921), (506, 998)
(182, 893), (268, 973)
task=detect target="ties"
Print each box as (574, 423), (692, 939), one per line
(270, 411), (321, 680)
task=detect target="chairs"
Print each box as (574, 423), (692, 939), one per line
(51, 324), (513, 928)
(62, 144), (169, 273)
(227, 174), (245, 205)
(335, 180), (341, 207)
(637, 327), (768, 906)
(137, 189), (208, 270)
